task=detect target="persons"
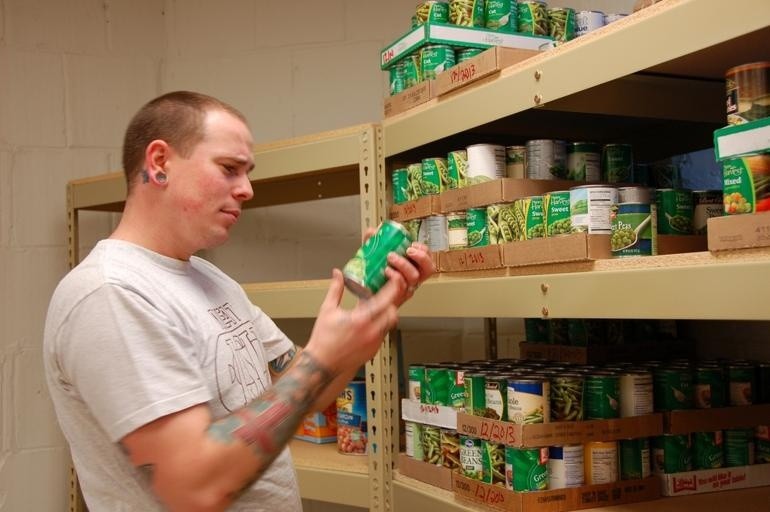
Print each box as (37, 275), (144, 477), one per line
(41, 90), (440, 512)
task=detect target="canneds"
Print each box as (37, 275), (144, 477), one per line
(726, 62), (769, 127)
(342, 220), (413, 299)
(391, 138), (723, 235)
(610, 203), (658, 257)
(388, 0), (628, 97)
(403, 356), (770, 493)
(337, 377), (376, 456)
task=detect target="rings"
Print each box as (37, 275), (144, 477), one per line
(408, 285), (419, 292)
